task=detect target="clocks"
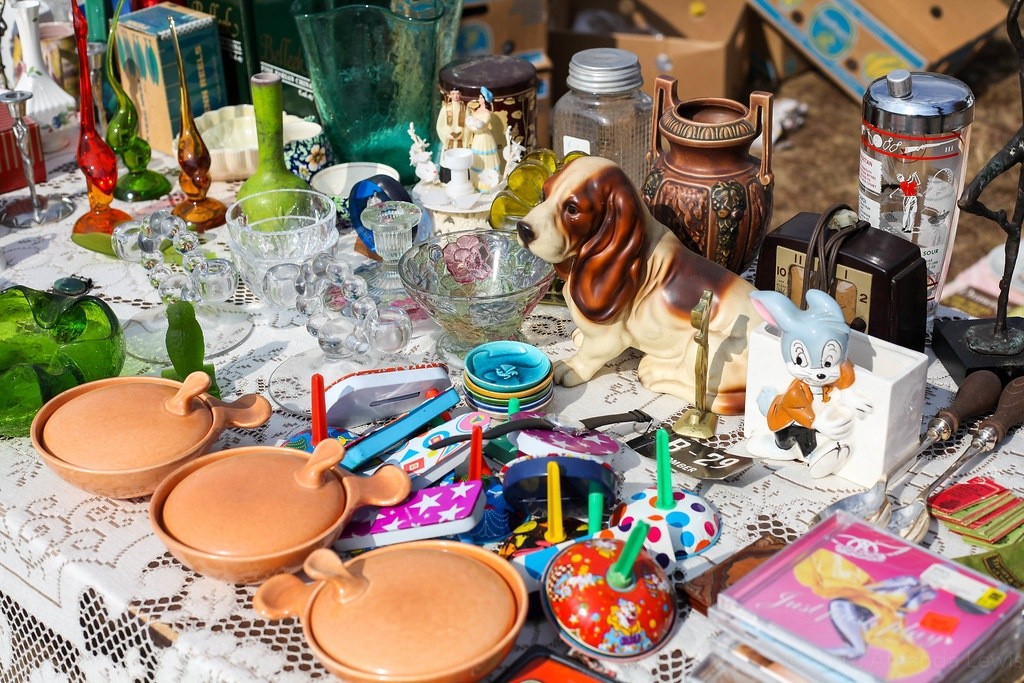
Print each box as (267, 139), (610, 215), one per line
(756, 205), (928, 353)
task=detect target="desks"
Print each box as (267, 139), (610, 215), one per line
(0, 162), (1024, 683)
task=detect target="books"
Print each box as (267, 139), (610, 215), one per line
(708, 509), (1024, 683)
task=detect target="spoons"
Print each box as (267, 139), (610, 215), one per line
(812, 370), (1002, 531)
(885, 376), (1024, 544)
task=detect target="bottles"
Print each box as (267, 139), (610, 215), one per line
(236, 72), (311, 233)
(552, 48), (654, 192)
(11, 1), (78, 160)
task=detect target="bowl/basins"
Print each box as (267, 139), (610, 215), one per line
(30, 371), (273, 499)
(282, 121), (335, 185)
(312, 162), (400, 224)
(252, 538), (528, 683)
(149, 438), (412, 587)
(225, 189), (339, 307)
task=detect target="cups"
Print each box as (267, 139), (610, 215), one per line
(397, 229), (558, 371)
(290, 0), (464, 185)
(858, 69), (976, 328)
(13, 21), (79, 100)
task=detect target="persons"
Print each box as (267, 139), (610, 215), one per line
(465, 93), (501, 194)
(436, 88), (468, 184)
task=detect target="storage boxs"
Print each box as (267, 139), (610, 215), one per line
(747, 0), (1010, 107)
(744, 320), (929, 487)
(112, 2), (225, 159)
(458, 0), (552, 151)
(544, 0), (746, 105)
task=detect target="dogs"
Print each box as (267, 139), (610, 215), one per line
(518, 156), (761, 417)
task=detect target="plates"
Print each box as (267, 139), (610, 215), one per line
(462, 341), (554, 420)
